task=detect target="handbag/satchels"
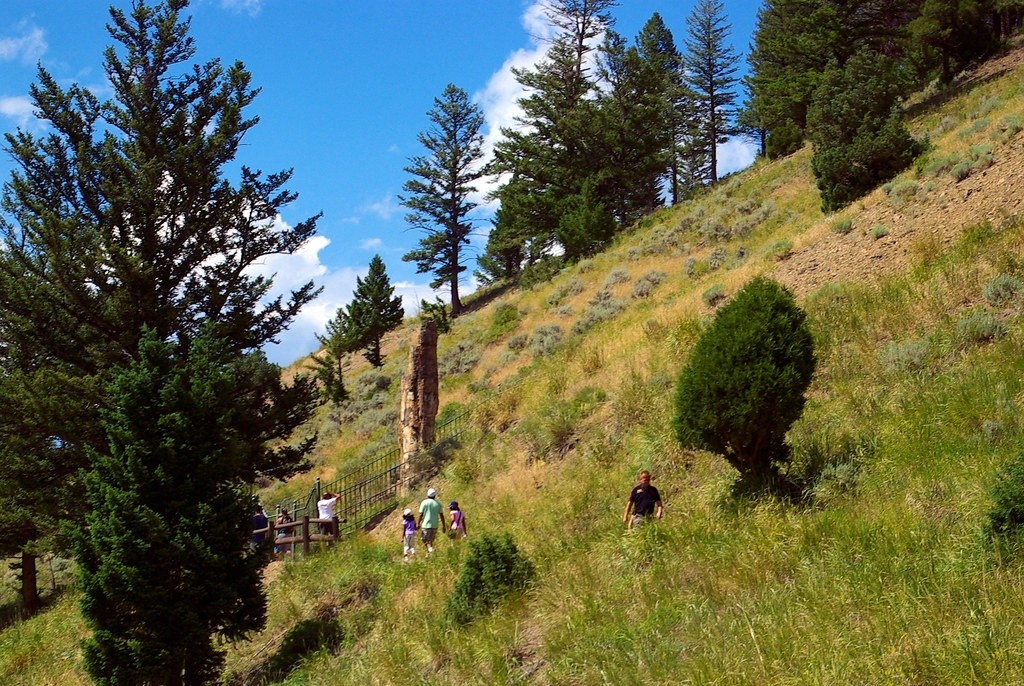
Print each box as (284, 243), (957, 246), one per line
(447, 529), (456, 539)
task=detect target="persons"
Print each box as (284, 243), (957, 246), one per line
(621, 470), (662, 530)
(416, 488), (446, 554)
(277, 509), (295, 561)
(250, 505), (267, 547)
(316, 490), (341, 536)
(450, 501), (468, 536)
(400, 508), (420, 561)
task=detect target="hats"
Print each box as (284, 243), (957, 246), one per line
(447, 501), (458, 509)
(427, 489), (436, 497)
(404, 509), (413, 515)
